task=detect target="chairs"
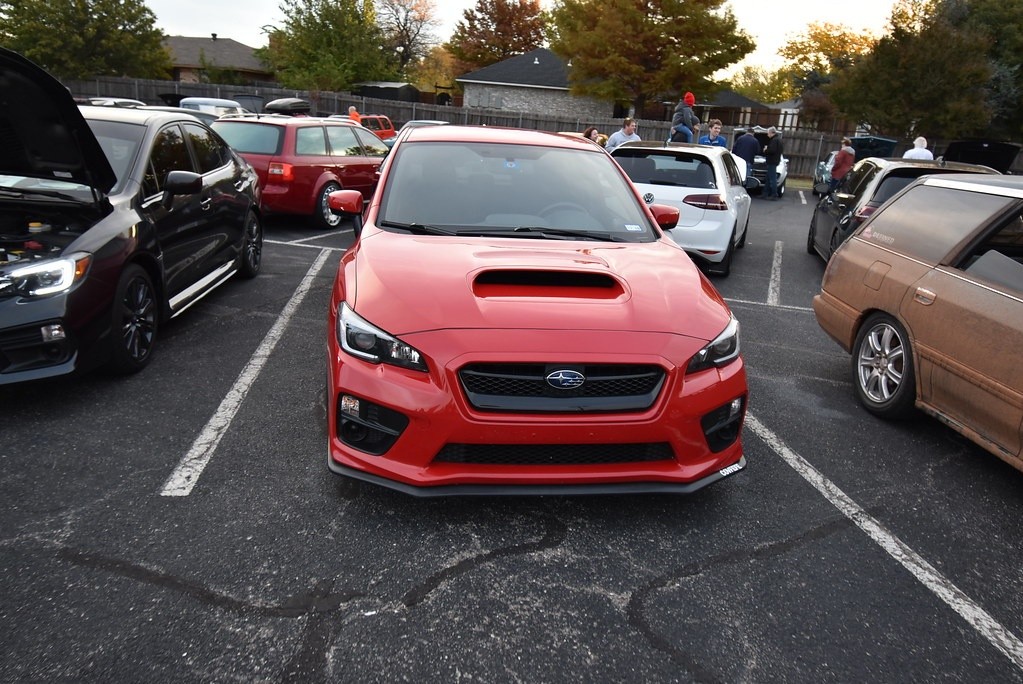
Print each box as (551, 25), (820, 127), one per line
(633, 158), (657, 180)
(539, 168), (608, 224)
(696, 163), (716, 187)
(398, 170), (489, 227)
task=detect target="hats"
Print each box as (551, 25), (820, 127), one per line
(912, 137), (928, 148)
(691, 116), (700, 127)
(684, 92), (695, 106)
(841, 137), (852, 145)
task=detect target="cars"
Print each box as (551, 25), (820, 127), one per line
(804, 154), (1023, 271)
(0, 43), (264, 387)
(322, 121), (748, 501)
(811, 172), (1023, 477)
(70, 93), (453, 148)
(811, 135), (899, 202)
(602, 138), (762, 281)
(729, 124), (790, 198)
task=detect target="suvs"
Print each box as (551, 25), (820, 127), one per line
(209, 112), (392, 230)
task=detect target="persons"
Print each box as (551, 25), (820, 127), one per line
(733, 128), (760, 176)
(671, 116), (700, 144)
(902, 136), (934, 159)
(698, 119), (727, 147)
(763, 126), (783, 200)
(584, 128), (599, 144)
(828, 137), (855, 193)
(605, 118), (642, 153)
(672, 92), (696, 145)
(349, 106), (361, 124)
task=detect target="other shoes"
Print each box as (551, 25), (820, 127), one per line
(765, 195), (778, 201)
(757, 192), (768, 199)
